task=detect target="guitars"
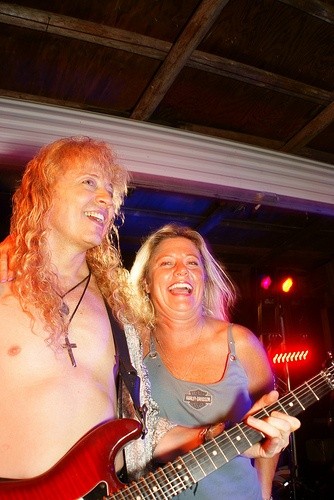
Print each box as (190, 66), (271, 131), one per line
(0, 353), (334, 500)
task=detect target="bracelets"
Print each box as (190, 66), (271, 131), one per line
(198, 425), (212, 446)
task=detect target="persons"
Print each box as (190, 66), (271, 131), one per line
(130, 223), (281, 500)
(0, 135), (300, 500)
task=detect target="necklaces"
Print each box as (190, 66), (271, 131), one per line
(49, 260), (92, 367)
(156, 319), (205, 380)
